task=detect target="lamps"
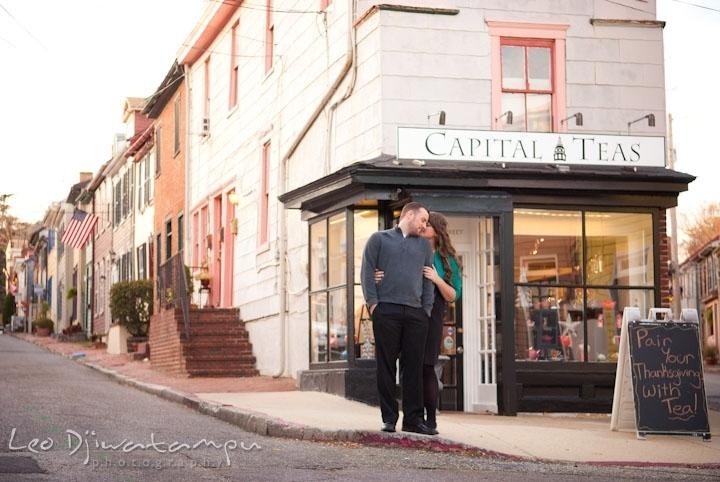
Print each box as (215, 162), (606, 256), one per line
(428, 110), (655, 127)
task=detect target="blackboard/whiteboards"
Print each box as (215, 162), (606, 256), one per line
(628, 322), (709, 434)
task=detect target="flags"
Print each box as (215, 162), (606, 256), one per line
(58, 208), (99, 251)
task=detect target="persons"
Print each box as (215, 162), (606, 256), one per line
(360, 199), (441, 435)
(369, 210), (463, 431)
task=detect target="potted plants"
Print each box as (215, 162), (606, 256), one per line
(32, 318), (51, 336)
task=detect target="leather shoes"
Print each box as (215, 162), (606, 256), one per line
(382, 424), (396, 432)
(402, 418), (436, 435)
